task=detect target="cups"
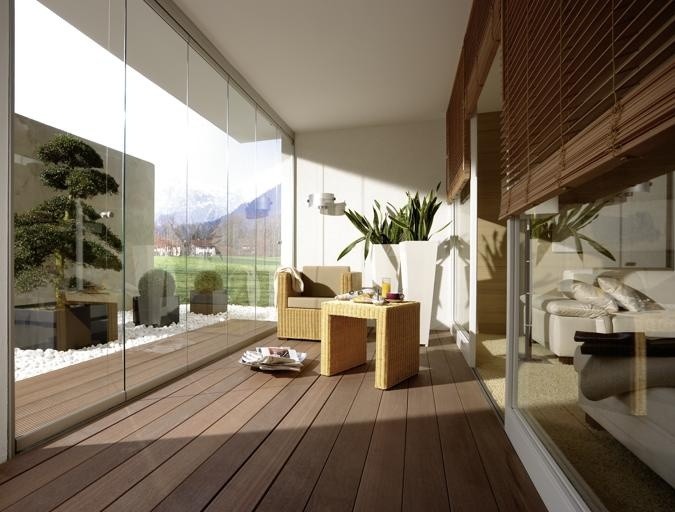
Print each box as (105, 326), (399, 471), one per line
(387, 292), (405, 300)
(381, 276), (392, 299)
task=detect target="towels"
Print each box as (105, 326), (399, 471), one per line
(273, 266), (304, 308)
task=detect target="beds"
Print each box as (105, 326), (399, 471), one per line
(573, 345), (675, 489)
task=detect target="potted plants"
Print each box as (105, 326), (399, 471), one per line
(190, 270), (228, 315)
(336, 180), (454, 348)
(132, 268), (180, 328)
(13, 132), (123, 351)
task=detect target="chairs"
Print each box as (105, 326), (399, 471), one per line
(276, 265), (363, 341)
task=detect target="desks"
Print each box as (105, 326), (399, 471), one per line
(320, 299), (420, 391)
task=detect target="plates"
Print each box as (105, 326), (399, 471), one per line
(384, 298), (402, 303)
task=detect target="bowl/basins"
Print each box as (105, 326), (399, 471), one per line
(372, 299), (385, 307)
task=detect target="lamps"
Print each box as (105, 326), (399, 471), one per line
(630, 180), (653, 192)
(317, 192), (334, 213)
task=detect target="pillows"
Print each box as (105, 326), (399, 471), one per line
(580, 355), (675, 401)
(517, 274), (656, 319)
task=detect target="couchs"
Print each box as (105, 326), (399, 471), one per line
(523, 266), (675, 360)
